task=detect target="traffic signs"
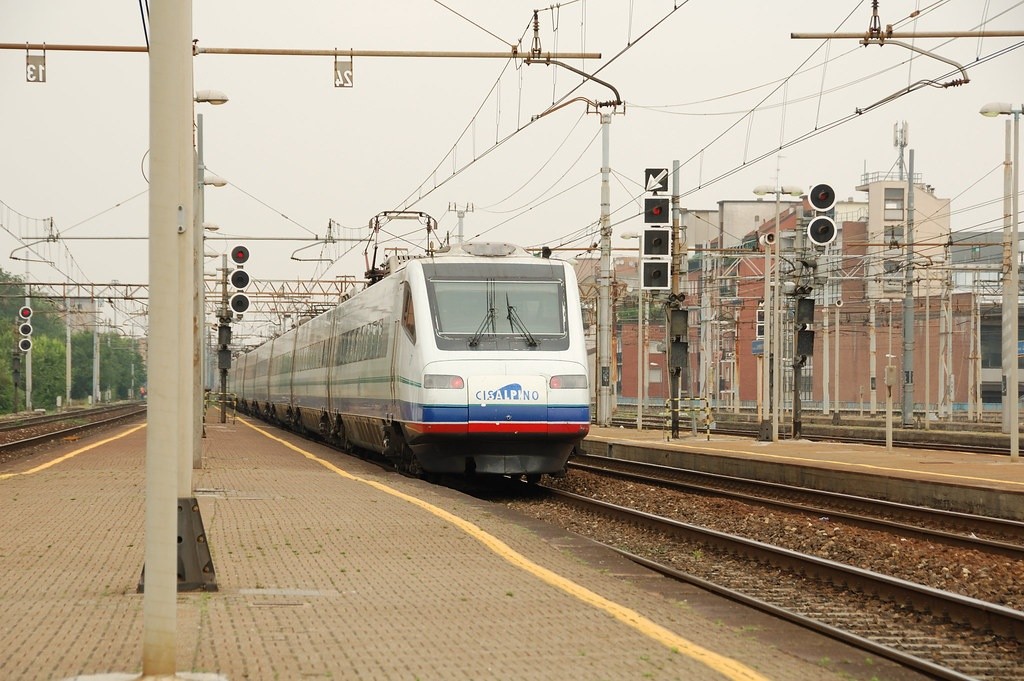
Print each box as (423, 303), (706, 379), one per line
(758, 300), (764, 308)
(645, 168), (668, 191)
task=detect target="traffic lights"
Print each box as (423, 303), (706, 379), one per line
(667, 309), (690, 369)
(217, 325), (232, 370)
(755, 310), (764, 337)
(12, 354), (21, 384)
(230, 246), (251, 316)
(18, 306), (33, 352)
(797, 299), (816, 356)
(799, 257), (827, 284)
(806, 181), (838, 247)
(641, 196), (674, 290)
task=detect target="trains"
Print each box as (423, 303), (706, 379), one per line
(227, 242), (592, 486)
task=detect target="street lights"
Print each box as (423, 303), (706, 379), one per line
(753, 184), (803, 445)
(621, 232), (643, 431)
(980, 101), (1024, 462)
